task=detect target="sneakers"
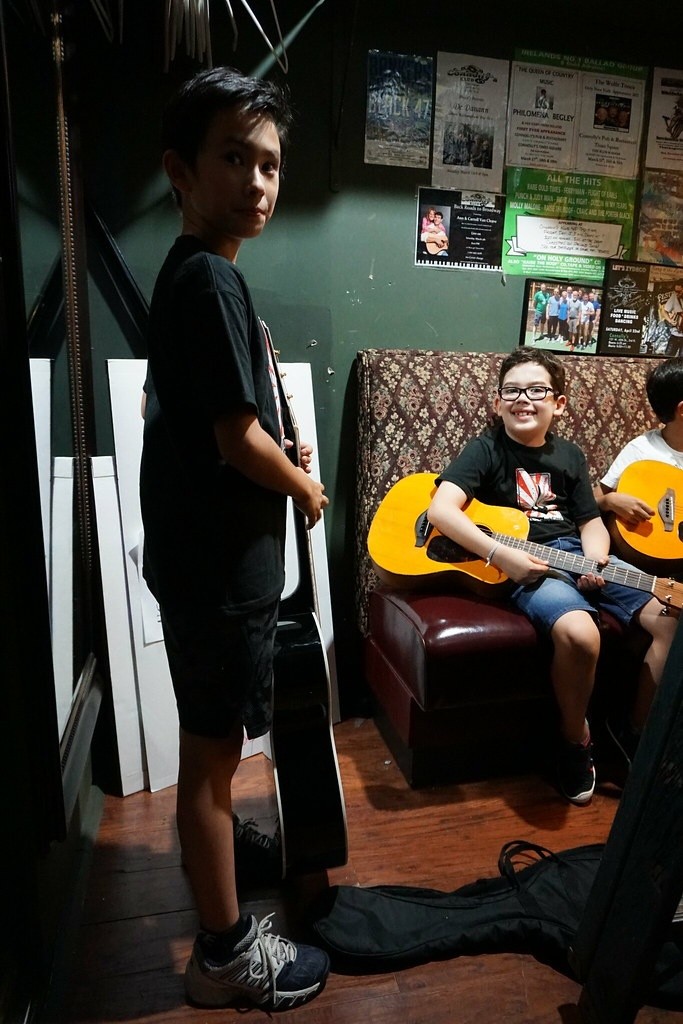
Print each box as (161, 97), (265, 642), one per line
(181, 912), (330, 1012)
(616, 713), (682, 789)
(180, 811), (283, 861)
(550, 703), (595, 804)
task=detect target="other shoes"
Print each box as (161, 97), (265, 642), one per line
(581, 345), (587, 350)
(556, 336), (564, 344)
(570, 345), (575, 351)
(566, 342), (571, 346)
(591, 338), (595, 343)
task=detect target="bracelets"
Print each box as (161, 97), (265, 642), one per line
(485, 542), (501, 568)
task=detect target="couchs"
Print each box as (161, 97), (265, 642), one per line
(352, 346), (667, 790)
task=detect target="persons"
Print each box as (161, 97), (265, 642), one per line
(421, 207), (448, 256)
(663, 280), (683, 356)
(471, 132), (493, 169)
(531, 282), (601, 351)
(594, 105), (629, 132)
(427, 346), (683, 803)
(135, 66), (332, 1011)
(537, 89), (550, 109)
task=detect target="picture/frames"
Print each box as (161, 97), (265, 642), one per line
(519, 279), (603, 356)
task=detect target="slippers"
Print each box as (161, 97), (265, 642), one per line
(498, 385), (555, 401)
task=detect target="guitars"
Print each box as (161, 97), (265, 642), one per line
(366, 471), (683, 617)
(260, 317), (351, 879)
(609, 459), (683, 569)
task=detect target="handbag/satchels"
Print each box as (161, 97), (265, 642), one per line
(673, 312), (683, 334)
